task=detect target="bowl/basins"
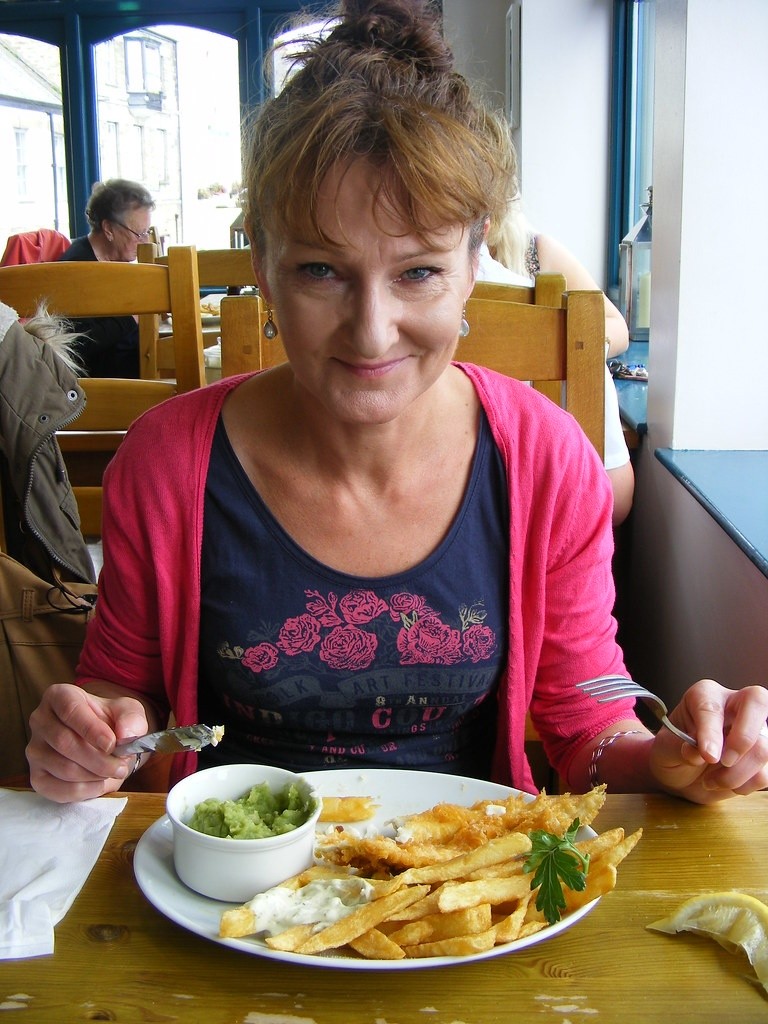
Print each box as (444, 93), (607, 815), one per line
(166, 763), (324, 904)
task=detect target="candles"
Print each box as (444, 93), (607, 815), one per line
(639, 269), (651, 327)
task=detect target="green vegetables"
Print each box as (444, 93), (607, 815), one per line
(500, 817), (592, 926)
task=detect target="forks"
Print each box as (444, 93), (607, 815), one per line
(575, 674), (700, 750)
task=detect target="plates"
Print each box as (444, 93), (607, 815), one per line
(199, 313), (221, 323)
(135, 769), (604, 969)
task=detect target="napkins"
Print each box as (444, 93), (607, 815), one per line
(0, 788), (129, 957)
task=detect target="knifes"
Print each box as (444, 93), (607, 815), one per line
(110, 724), (216, 758)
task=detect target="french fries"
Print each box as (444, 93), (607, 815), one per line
(219, 784), (646, 960)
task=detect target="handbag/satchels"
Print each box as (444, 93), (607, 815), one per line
(0, 550), (101, 779)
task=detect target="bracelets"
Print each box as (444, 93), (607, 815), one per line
(587, 730), (652, 790)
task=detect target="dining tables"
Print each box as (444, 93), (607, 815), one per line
(0, 785), (768, 1024)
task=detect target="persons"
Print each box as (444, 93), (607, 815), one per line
(24, 1), (767, 805)
(56, 179), (154, 378)
(476, 211), (636, 527)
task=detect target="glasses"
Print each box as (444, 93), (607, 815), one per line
(107, 218), (153, 242)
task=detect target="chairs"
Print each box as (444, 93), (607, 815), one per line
(1, 237), (607, 537)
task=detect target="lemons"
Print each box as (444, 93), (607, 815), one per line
(646, 893), (768, 996)
